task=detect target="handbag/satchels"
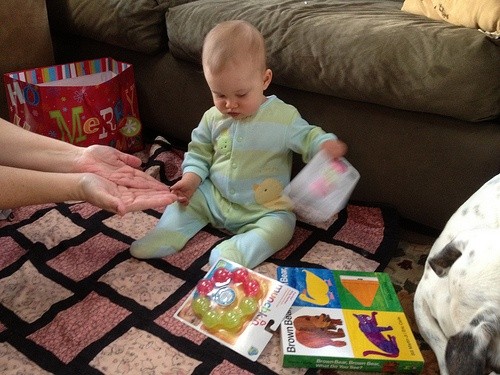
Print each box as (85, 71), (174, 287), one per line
(3, 57), (144, 153)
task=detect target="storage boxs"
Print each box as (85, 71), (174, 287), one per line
(276, 266), (425, 375)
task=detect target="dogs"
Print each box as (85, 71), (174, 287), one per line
(414, 172), (500, 375)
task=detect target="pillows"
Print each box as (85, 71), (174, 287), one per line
(401, 0), (500, 35)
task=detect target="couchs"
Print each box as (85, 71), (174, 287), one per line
(45, 0), (500, 239)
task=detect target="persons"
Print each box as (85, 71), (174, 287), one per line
(131, 21), (347, 269)
(0, 118), (178, 216)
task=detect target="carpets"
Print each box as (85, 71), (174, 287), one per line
(0, 135), (405, 375)
